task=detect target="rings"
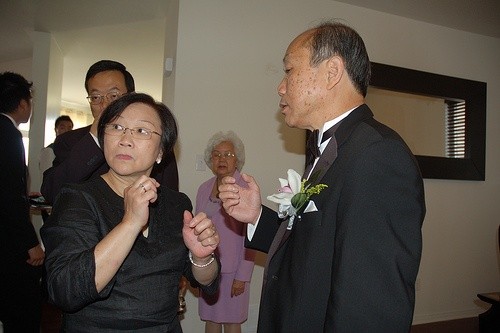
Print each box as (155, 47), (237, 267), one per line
(208, 224), (216, 235)
(140, 184), (147, 192)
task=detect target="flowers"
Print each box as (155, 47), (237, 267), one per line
(266, 167), (330, 232)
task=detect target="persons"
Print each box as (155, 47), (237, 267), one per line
(0, 60), (179, 333)
(195, 130), (256, 333)
(218, 21), (426, 333)
(40, 93), (221, 333)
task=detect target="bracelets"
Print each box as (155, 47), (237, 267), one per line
(188, 250), (215, 268)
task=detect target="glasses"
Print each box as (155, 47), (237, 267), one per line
(101, 124), (163, 139)
(87, 92), (127, 105)
(211, 151), (237, 160)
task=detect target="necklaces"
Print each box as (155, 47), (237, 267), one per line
(216, 178), (220, 198)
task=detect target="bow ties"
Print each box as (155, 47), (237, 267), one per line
(304, 129), (321, 170)
